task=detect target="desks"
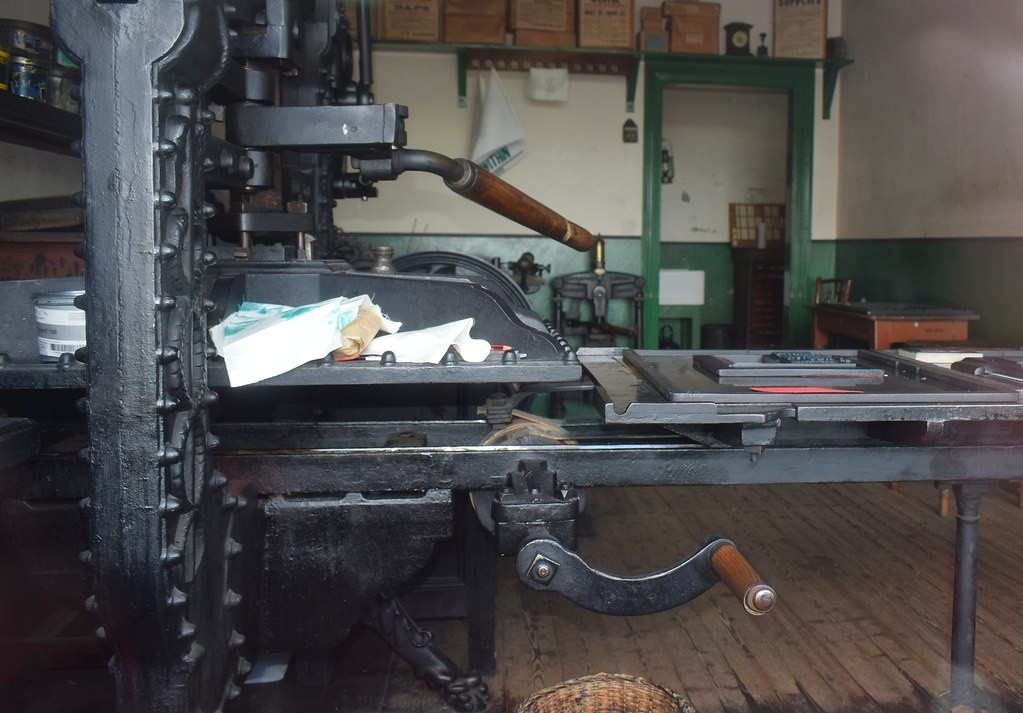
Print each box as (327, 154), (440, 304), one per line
(659, 305), (702, 349)
(805, 303), (981, 349)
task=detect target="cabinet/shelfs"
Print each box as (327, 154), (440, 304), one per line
(734, 247), (784, 349)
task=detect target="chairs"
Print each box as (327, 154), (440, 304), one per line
(816, 277), (852, 304)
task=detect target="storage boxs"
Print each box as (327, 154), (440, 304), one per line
(344, 0), (634, 50)
(636, 0), (720, 54)
(773, 0), (826, 59)
(659, 269), (705, 306)
